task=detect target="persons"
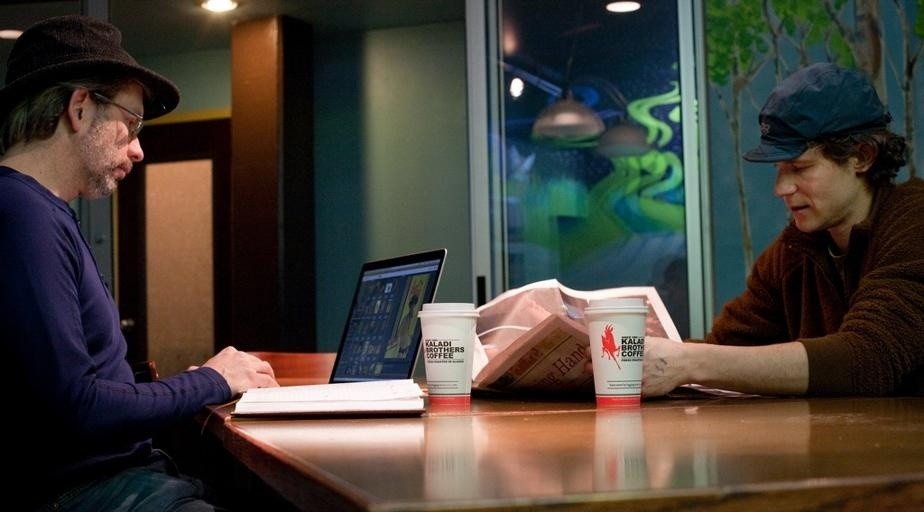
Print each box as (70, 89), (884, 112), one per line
(579, 60), (922, 400)
(385, 294), (419, 359)
(0, 16), (284, 512)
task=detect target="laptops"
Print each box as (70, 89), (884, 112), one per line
(238, 247), (448, 401)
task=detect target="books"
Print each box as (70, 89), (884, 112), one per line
(225, 377), (428, 418)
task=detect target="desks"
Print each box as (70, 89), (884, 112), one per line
(200, 396), (924, 512)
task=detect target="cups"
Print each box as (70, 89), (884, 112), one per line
(585, 295), (652, 410)
(416, 299), (481, 417)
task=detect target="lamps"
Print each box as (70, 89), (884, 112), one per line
(531, 35), (652, 160)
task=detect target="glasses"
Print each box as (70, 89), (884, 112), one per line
(80, 90), (145, 141)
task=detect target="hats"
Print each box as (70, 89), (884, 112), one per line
(743, 63), (890, 162)
(6, 14), (180, 120)
